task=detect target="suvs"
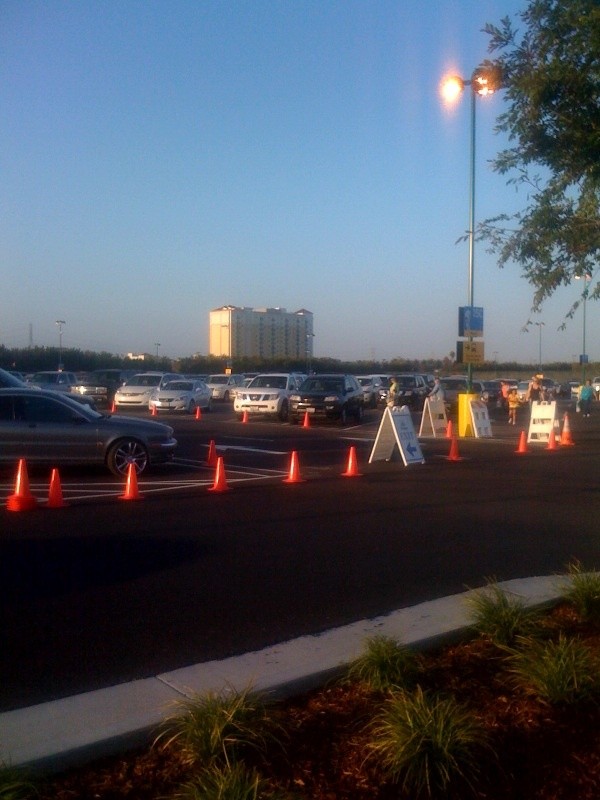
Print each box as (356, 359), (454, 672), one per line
(287, 373), (367, 425)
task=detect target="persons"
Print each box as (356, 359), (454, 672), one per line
(386, 377), (600, 426)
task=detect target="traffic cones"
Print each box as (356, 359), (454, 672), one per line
(43, 467), (70, 510)
(120, 462), (146, 500)
(300, 411), (312, 428)
(240, 409), (249, 423)
(204, 438), (220, 468)
(196, 405), (201, 421)
(205, 455), (232, 492)
(6, 458), (39, 514)
(444, 418), (458, 439)
(515, 430), (530, 453)
(281, 449), (309, 483)
(341, 447), (365, 477)
(110, 398), (117, 415)
(151, 402), (160, 417)
(444, 434), (468, 463)
(557, 414), (576, 447)
(542, 428), (562, 449)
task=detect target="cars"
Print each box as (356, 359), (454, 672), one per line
(0, 388), (180, 483)
(0, 370), (600, 420)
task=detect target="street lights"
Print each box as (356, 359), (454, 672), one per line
(574, 269), (593, 386)
(303, 332), (316, 379)
(441, 67), (502, 396)
(153, 341), (163, 369)
(52, 320), (68, 371)
